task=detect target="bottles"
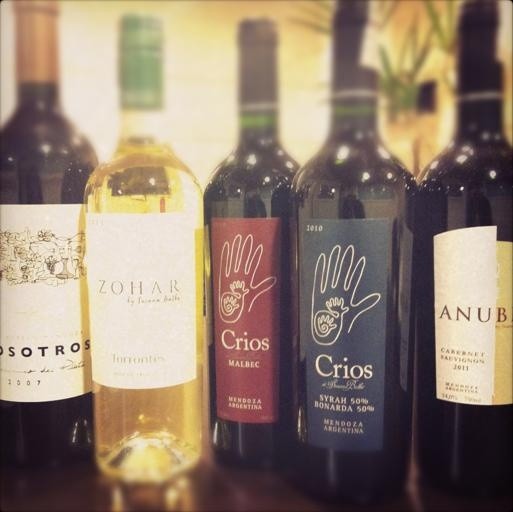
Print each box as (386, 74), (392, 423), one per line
(79, 13), (210, 481)
(203, 18), (300, 469)
(0, 0), (100, 492)
(410, 59), (513, 512)
(289, 64), (415, 512)
